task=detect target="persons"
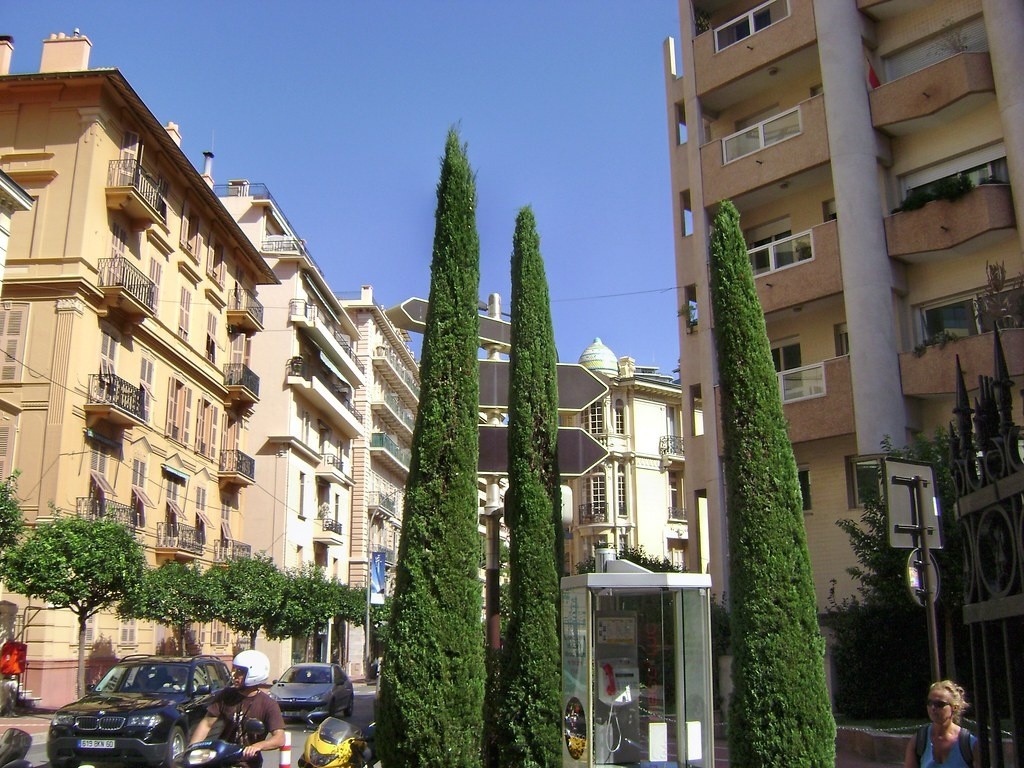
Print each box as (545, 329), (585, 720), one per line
(319, 671), (327, 680)
(903, 680), (980, 768)
(137, 671), (149, 689)
(188, 650), (286, 768)
(176, 668), (187, 685)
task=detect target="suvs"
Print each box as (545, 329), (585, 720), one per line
(46, 654), (234, 768)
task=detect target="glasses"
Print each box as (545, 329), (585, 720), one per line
(924, 698), (950, 709)
(236, 668), (246, 674)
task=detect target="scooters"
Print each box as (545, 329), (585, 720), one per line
(172, 718), (265, 768)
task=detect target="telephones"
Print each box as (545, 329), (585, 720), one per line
(597, 657), (641, 706)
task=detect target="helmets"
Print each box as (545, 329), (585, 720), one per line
(232, 651), (270, 687)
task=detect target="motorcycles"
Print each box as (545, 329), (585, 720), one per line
(298, 710), (376, 768)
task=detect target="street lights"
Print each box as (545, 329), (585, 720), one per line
(366, 484), (406, 682)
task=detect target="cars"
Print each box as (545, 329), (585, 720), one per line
(268, 663), (354, 723)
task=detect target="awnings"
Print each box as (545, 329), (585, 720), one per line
(91, 469), (118, 497)
(221, 521), (233, 539)
(131, 484), (157, 509)
(165, 497), (188, 521)
(196, 508), (215, 530)
